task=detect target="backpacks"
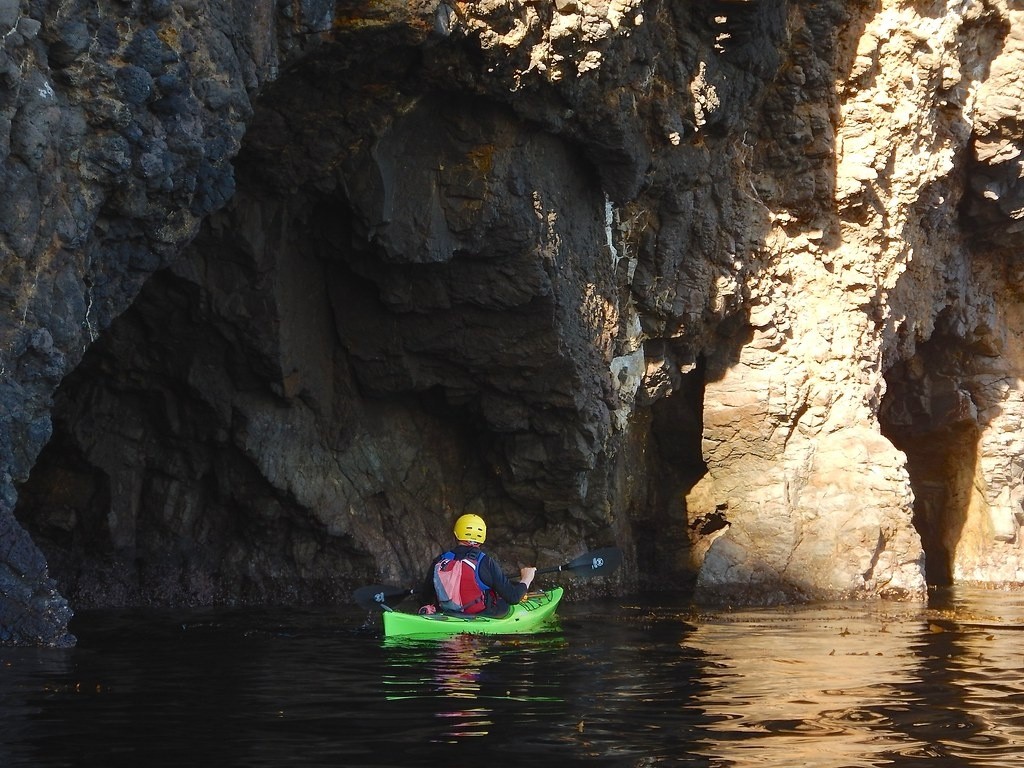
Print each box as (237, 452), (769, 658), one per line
(432, 547), (497, 616)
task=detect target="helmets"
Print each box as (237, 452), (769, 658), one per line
(453, 514), (486, 544)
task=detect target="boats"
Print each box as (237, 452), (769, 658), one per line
(383, 586), (563, 636)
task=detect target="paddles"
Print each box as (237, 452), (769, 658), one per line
(353, 546), (623, 614)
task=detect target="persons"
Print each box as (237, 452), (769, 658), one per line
(413, 514), (538, 618)
